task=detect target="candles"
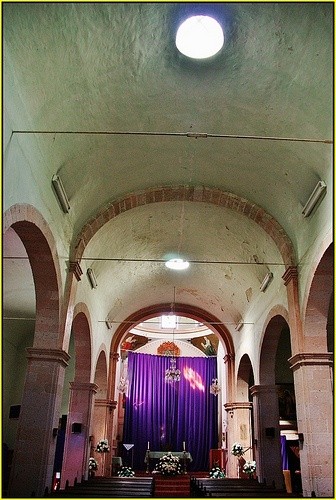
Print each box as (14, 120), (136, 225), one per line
(148, 441), (149, 449)
(183, 441), (185, 450)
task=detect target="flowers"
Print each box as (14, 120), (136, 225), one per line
(88, 458), (98, 471)
(230, 443), (244, 456)
(151, 452), (186, 475)
(242, 460), (256, 474)
(95, 440), (110, 453)
(209, 467), (225, 479)
(117, 466), (135, 477)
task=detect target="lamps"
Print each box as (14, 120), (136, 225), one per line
(117, 377), (129, 394)
(210, 378), (222, 396)
(165, 329), (181, 383)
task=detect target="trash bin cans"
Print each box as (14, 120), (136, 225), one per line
(112, 457), (122, 477)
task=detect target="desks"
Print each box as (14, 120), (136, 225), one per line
(144, 452), (193, 474)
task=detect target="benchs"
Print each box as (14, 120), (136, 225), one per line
(190, 477), (316, 498)
(30, 471), (155, 498)
